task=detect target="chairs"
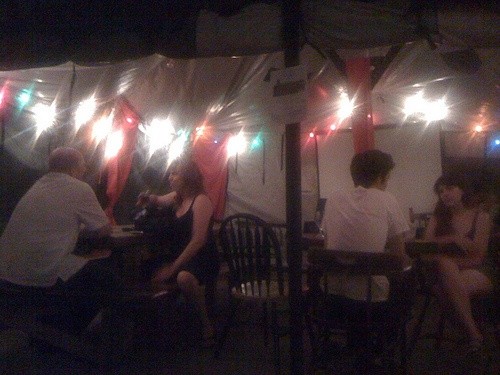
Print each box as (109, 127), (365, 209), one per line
(211, 210), (426, 375)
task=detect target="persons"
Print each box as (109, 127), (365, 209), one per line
(421, 167), (499, 362)
(135, 158), (218, 349)
(0, 147), (113, 351)
(320, 150), (418, 375)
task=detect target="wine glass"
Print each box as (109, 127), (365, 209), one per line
(315, 211), (326, 238)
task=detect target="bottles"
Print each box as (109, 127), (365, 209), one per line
(406, 208), (417, 240)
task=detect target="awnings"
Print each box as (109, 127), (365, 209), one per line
(0, 42), (500, 151)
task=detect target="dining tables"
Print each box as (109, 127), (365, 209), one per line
(295, 233), (439, 375)
(76, 225), (168, 368)
